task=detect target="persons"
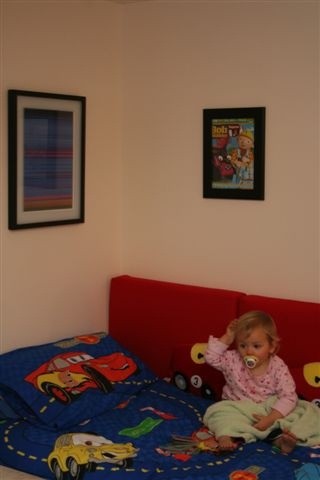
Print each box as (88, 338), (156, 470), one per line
(202, 309), (320, 456)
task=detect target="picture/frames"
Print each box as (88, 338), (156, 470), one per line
(202, 107), (267, 201)
(7, 89), (87, 230)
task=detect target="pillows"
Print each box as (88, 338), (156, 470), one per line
(0, 331), (156, 430)
